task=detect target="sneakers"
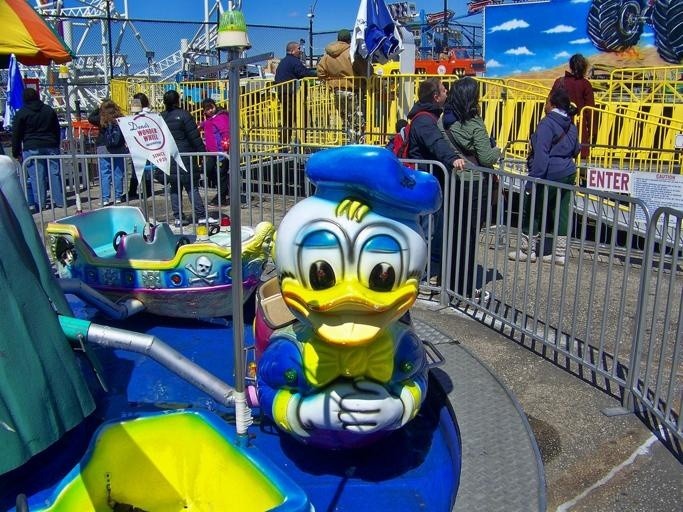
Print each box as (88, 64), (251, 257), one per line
(196, 215), (219, 226)
(175, 218), (190, 227)
(461, 287), (490, 303)
(97, 196), (122, 207)
(209, 192), (228, 207)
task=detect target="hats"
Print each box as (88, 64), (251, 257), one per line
(338, 29), (351, 42)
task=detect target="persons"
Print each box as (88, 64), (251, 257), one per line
(545, 55), (597, 164)
(317, 29), (367, 145)
(404, 78), (469, 296)
(12, 87), (67, 211)
(196, 99), (230, 206)
(158, 91), (219, 228)
(121, 93), (154, 203)
(88, 96), (126, 207)
(438, 79), (500, 303)
(507, 91), (584, 267)
(274, 42), (317, 158)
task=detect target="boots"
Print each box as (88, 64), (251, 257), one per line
(508, 230), (541, 263)
(541, 233), (568, 266)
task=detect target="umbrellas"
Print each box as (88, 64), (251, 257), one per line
(0, 0), (73, 68)
(2, 53), (26, 134)
(349, 0), (404, 90)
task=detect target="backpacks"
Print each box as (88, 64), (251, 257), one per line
(104, 123), (125, 147)
(387, 119), (416, 170)
